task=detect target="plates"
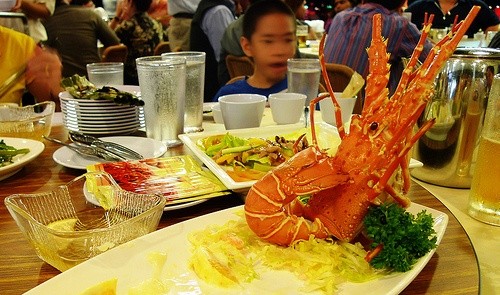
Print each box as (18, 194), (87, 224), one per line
(52, 136), (168, 169)
(82, 167), (209, 211)
(177, 120), (424, 192)
(203, 101), (216, 120)
(22, 198), (449, 295)
(0, 136), (46, 180)
(59, 84), (145, 136)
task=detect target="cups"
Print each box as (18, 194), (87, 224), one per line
(466, 74), (500, 226)
(433, 29), (447, 41)
(211, 103), (223, 125)
(268, 93), (307, 125)
(473, 30), (497, 47)
(136, 54), (187, 148)
(161, 51), (206, 132)
(318, 93), (357, 126)
(296, 25), (308, 48)
(286, 58), (321, 121)
(218, 94), (267, 130)
(86, 62), (124, 84)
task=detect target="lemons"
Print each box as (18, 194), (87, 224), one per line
(192, 246), (241, 287)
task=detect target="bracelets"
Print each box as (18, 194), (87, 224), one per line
(115, 17), (120, 21)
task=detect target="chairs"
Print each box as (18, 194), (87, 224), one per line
(103, 43), (362, 114)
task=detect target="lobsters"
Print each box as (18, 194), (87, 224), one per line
(245, 4), (482, 249)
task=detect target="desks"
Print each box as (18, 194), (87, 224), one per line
(300, 37), (500, 73)
(0, 102), (500, 295)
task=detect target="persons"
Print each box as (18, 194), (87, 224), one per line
(405, 0), (500, 38)
(168, 0), (251, 100)
(215, 0), (297, 101)
(0, 26), (64, 111)
(12, 0), (55, 47)
(48, 0), (120, 76)
(108, 0), (170, 85)
(323, 0), (435, 99)
(285, 0), (355, 33)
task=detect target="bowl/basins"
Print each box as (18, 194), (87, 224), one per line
(4, 170), (166, 273)
(0, 100), (55, 141)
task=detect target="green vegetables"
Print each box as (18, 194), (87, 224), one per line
(0, 139), (30, 169)
(364, 200), (440, 273)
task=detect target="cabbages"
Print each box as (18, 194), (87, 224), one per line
(186, 208), (383, 295)
(199, 131), (275, 171)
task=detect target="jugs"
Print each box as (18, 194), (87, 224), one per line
(403, 47), (500, 189)
